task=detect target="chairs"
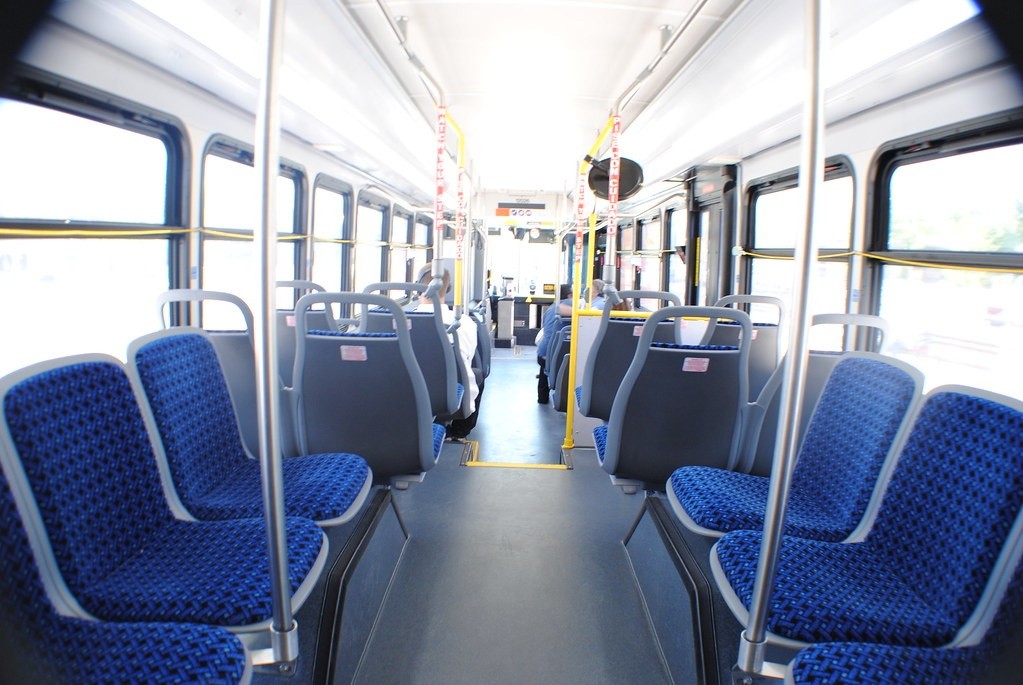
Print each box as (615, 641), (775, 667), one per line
(0, 268), (1022, 685)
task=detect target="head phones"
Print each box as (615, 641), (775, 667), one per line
(415, 262), (452, 295)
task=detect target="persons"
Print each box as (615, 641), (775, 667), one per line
(533, 278), (625, 404)
(407, 260), (486, 445)
